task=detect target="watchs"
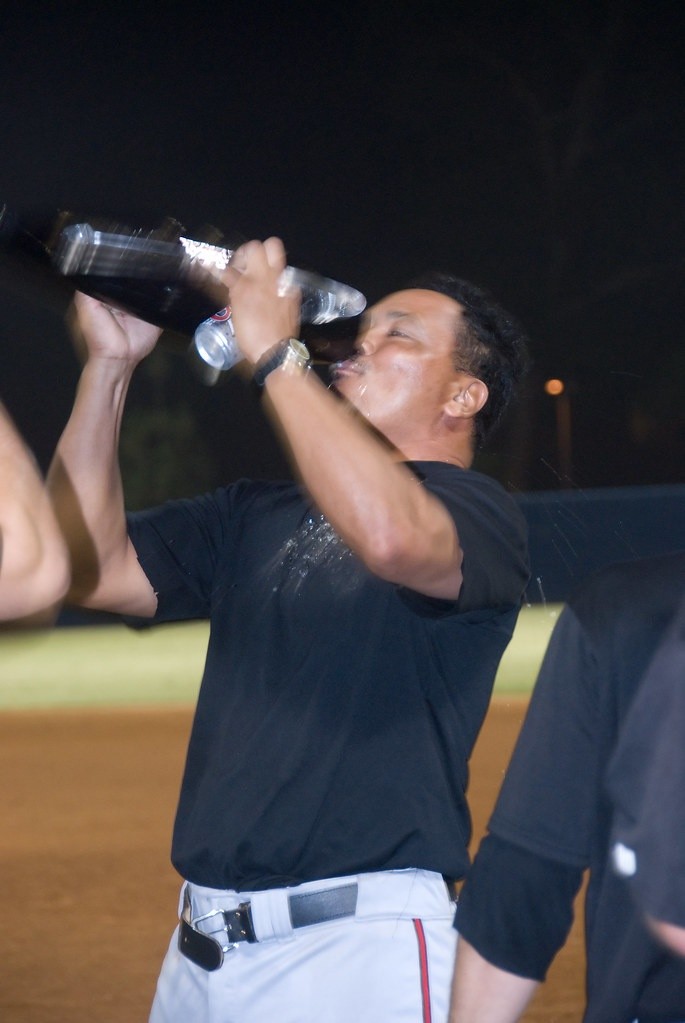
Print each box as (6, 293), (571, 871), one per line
(249, 338), (315, 400)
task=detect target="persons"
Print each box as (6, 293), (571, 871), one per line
(0, 406), (71, 622)
(450, 550), (685, 1022)
(48, 236), (531, 1023)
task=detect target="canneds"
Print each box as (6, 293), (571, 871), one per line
(195, 303), (246, 371)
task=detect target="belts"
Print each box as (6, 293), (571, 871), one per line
(178, 883), (357, 972)
